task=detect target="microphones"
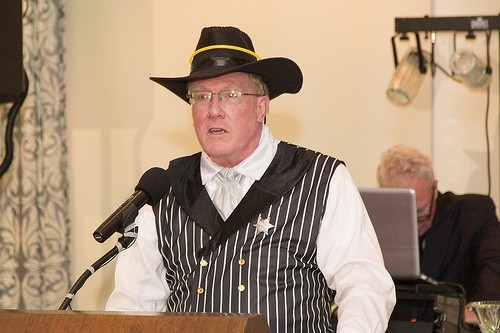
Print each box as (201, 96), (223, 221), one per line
(92, 167), (170, 243)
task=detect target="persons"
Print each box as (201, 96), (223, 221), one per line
(377, 143), (500, 333)
(107, 26), (396, 333)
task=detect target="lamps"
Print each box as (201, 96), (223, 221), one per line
(385, 31), (431, 107)
(448, 29), (492, 92)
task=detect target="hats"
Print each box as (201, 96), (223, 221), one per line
(148, 27), (304, 104)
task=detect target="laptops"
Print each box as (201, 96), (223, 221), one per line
(359, 189), (438, 284)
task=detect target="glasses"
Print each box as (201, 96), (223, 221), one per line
(185, 90), (264, 104)
(417, 184), (436, 223)
